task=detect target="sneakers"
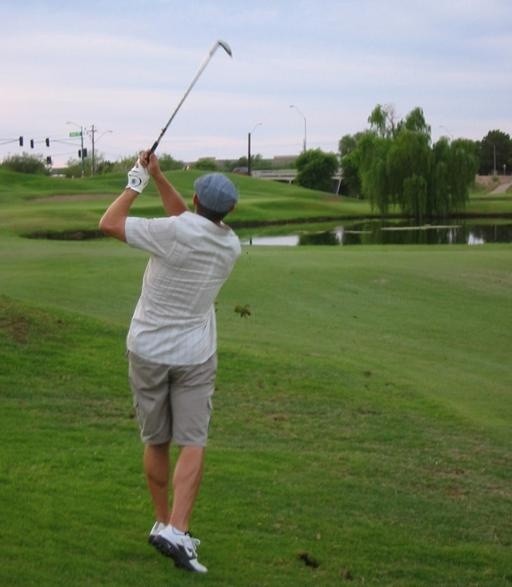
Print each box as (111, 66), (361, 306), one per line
(148, 521), (207, 572)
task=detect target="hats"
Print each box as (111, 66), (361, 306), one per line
(194, 172), (237, 212)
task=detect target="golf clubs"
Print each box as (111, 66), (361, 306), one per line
(145, 40), (232, 165)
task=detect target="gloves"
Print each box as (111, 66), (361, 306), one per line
(128, 158), (150, 192)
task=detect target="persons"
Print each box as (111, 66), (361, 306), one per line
(98, 150), (242, 574)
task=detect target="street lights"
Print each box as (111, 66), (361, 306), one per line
(69, 132), (82, 137)
(290, 104), (306, 153)
(66, 121), (85, 179)
(248, 123), (263, 175)
(91, 130), (114, 176)
